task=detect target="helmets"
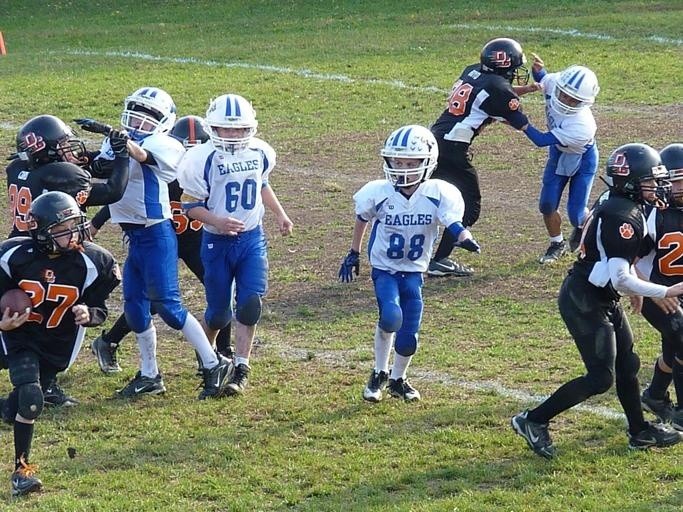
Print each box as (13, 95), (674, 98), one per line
(480, 38), (529, 86)
(122, 88), (176, 142)
(658, 143), (682, 209)
(551, 66), (599, 116)
(382, 125), (439, 187)
(606, 144), (672, 211)
(17, 116), (88, 166)
(173, 115), (206, 148)
(203, 94), (257, 155)
(27, 192), (93, 254)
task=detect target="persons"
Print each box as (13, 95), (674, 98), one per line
(0, 85), (294, 497)
(339, 125), (481, 403)
(427, 39), (682, 459)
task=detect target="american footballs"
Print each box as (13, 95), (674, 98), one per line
(0, 289), (33, 317)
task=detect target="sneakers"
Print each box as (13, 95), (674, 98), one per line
(511, 410), (554, 459)
(9, 473), (39, 495)
(670, 409), (682, 430)
(364, 368), (389, 401)
(642, 390), (675, 421)
(540, 238), (566, 264)
(387, 377), (421, 403)
(42, 384), (79, 408)
(569, 225), (581, 253)
(92, 330), (120, 374)
(428, 257), (473, 277)
(625, 421), (682, 450)
(194, 346), (249, 399)
(115, 370), (166, 397)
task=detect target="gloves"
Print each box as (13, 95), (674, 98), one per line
(110, 130), (130, 158)
(453, 239), (479, 254)
(337, 249), (359, 282)
(74, 117), (113, 137)
